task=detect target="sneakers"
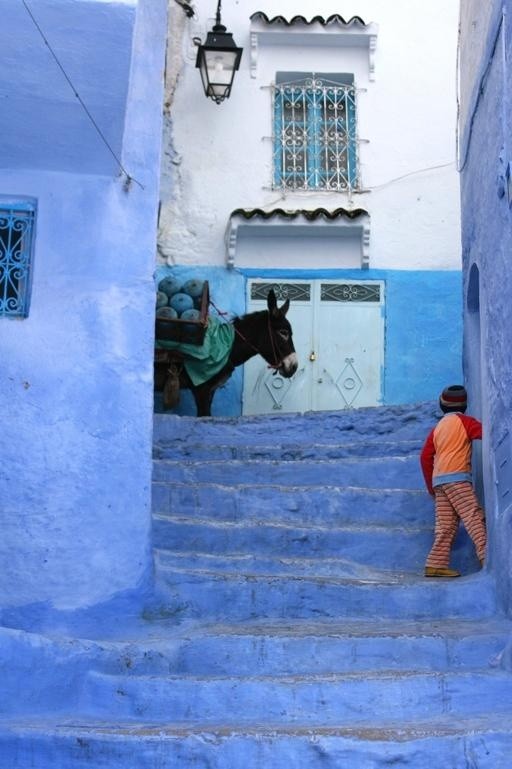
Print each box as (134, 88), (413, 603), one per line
(425, 562), (460, 579)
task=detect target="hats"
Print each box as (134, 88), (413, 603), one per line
(439, 384), (467, 413)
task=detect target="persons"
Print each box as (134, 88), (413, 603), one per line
(419, 384), (487, 578)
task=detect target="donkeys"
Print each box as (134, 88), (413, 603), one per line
(154, 287), (300, 419)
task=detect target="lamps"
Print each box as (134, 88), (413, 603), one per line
(192, 0), (247, 109)
